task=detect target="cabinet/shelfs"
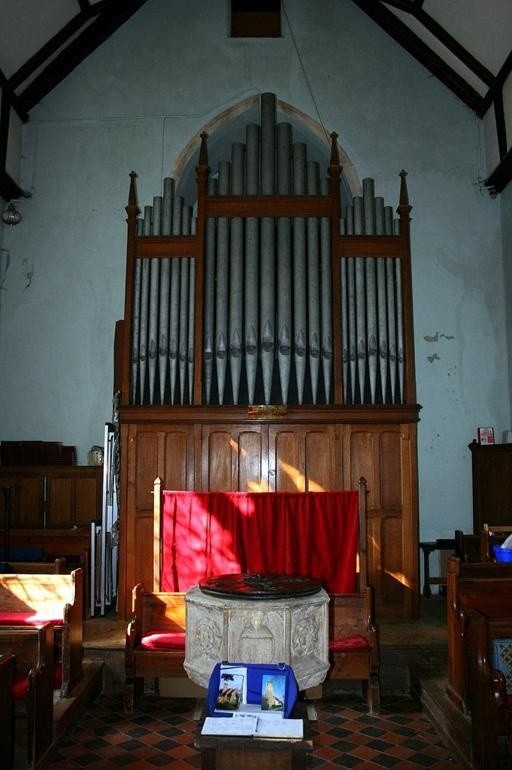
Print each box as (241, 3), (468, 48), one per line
(0, 465), (104, 620)
(115, 404), (423, 624)
(419, 524), (512, 599)
(467, 438), (511, 536)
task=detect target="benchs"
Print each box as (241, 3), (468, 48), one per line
(0, 568), (85, 699)
(122, 476), (381, 715)
(447, 555), (511, 769)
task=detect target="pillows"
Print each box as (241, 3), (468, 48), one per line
(491, 639), (511, 695)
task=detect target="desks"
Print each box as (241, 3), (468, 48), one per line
(2, 626), (54, 764)
